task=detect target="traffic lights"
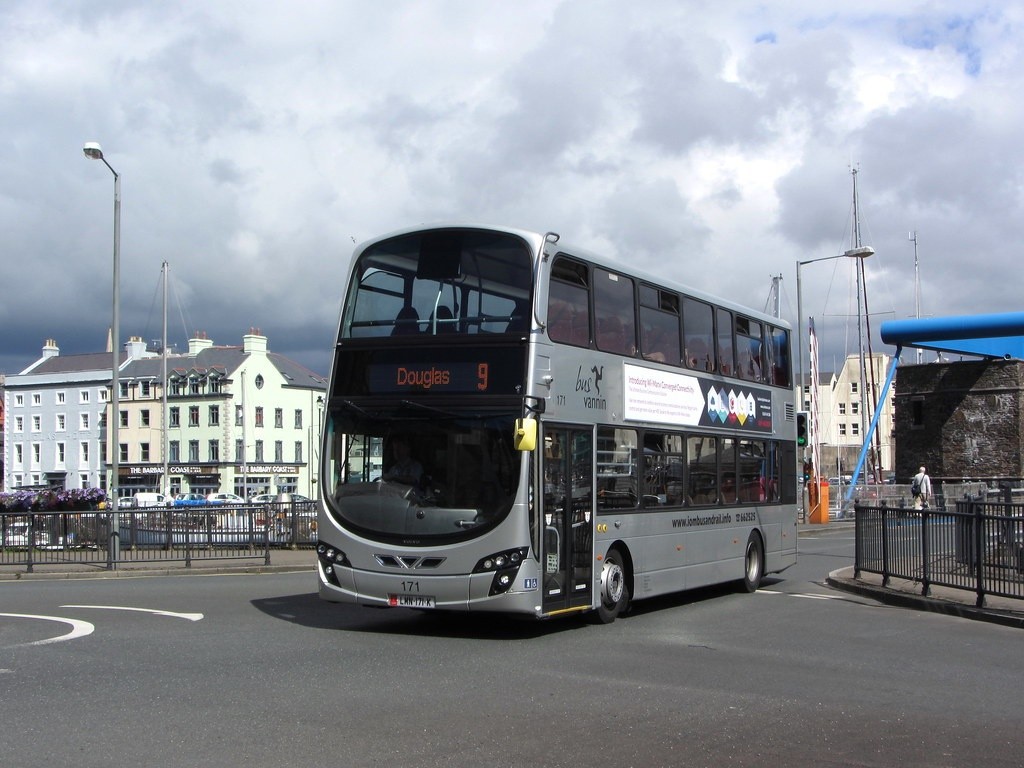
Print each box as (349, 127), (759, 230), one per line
(797, 414), (806, 447)
(803, 461), (811, 479)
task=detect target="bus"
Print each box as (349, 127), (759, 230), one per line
(315, 224), (795, 624)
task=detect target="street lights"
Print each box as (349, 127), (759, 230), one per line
(82, 142), (121, 570)
(796, 245), (876, 524)
(316, 394), (323, 445)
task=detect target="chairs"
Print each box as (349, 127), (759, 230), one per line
(686, 476), (774, 505)
(388, 298), (778, 386)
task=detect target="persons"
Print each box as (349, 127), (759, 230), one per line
(911, 467), (932, 518)
(383, 437), (424, 486)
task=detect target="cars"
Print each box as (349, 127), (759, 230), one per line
(111, 491), (317, 516)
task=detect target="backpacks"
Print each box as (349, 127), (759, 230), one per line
(911, 485), (921, 498)
